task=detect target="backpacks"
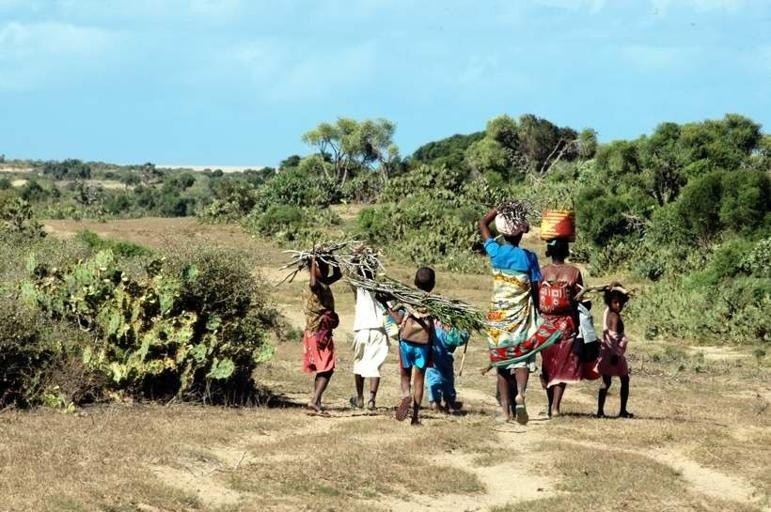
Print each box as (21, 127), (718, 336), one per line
(539, 280), (574, 314)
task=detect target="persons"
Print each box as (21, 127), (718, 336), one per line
(591, 282), (636, 418)
(532, 207), (600, 418)
(373, 267), (451, 426)
(301, 242), (343, 417)
(426, 295), (474, 417)
(345, 241), (394, 411)
(478, 198), (545, 425)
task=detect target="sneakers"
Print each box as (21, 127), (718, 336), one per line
(445, 401), (462, 410)
(432, 402), (444, 411)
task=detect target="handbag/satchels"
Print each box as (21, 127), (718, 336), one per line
(320, 310), (339, 328)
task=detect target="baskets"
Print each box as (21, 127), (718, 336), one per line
(540, 198), (576, 242)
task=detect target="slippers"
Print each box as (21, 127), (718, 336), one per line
(396, 396), (411, 421)
(517, 405), (528, 424)
(410, 420), (424, 426)
(350, 396), (364, 409)
(504, 415), (511, 423)
(367, 400), (376, 410)
(307, 406), (331, 418)
